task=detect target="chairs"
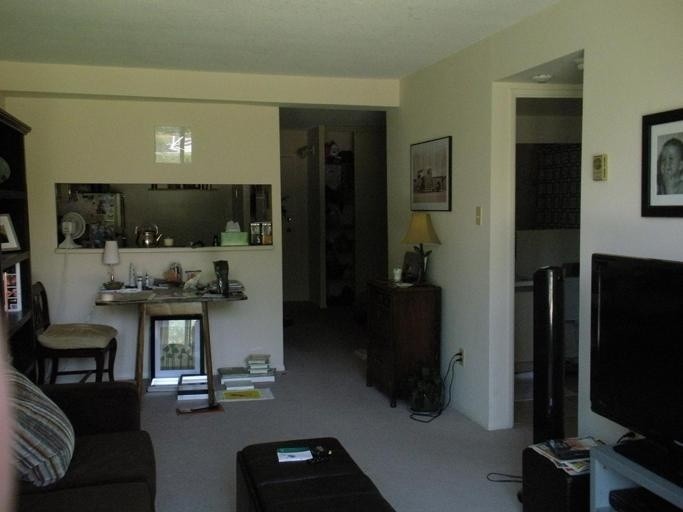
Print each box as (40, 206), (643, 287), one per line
(12, 380), (156, 511)
(30, 280), (117, 384)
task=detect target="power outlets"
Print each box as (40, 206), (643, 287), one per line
(457, 348), (465, 366)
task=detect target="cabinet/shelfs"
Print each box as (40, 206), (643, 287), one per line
(0, 108), (37, 384)
(588, 437), (682, 512)
(365, 279), (442, 407)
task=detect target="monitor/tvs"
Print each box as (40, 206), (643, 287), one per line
(590, 253), (683, 490)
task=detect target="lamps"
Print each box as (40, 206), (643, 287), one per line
(398, 212), (443, 287)
(101, 240), (123, 290)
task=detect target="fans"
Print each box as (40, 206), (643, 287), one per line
(57, 211), (86, 249)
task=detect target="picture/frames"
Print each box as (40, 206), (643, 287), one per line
(150, 313), (204, 380)
(640, 106), (683, 219)
(408, 136), (452, 212)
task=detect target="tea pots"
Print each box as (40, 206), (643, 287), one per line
(133, 222), (164, 247)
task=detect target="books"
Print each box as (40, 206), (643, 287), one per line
(527, 434), (607, 475)
(176, 354), (277, 401)
(207, 278), (248, 296)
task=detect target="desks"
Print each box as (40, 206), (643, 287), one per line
(92, 284), (248, 407)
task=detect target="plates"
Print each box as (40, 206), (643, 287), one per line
(393, 282), (414, 288)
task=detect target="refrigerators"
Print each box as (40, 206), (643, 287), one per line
(74, 193), (126, 231)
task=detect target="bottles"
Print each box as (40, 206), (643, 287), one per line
(137, 276), (142, 289)
(145, 273), (150, 287)
(164, 236), (174, 247)
(213, 235), (219, 246)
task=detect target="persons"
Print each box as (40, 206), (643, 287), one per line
(656, 137), (683, 194)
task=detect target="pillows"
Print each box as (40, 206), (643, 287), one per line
(1, 360), (76, 487)
